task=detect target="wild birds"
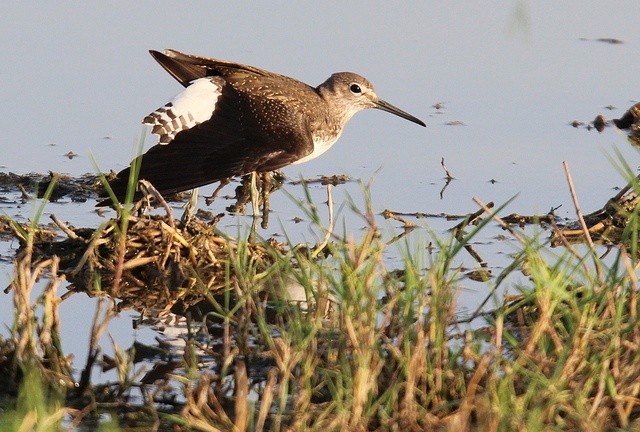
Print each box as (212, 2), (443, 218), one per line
(94, 47), (426, 229)
